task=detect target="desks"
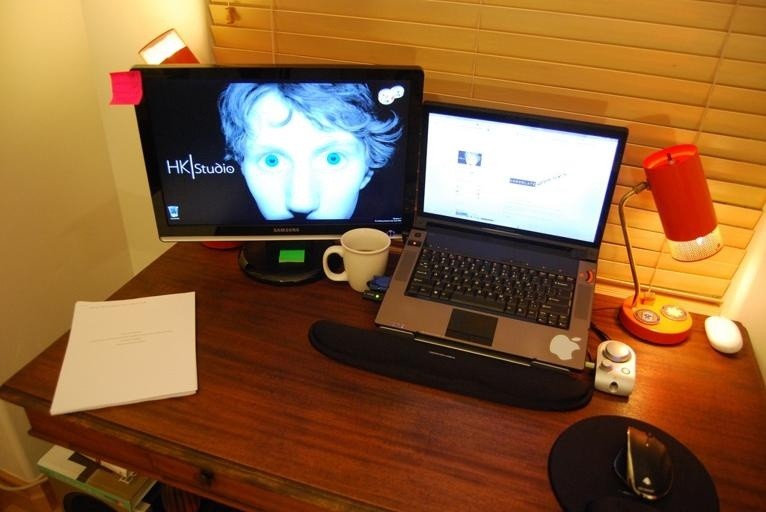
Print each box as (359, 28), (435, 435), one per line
(1, 238), (766, 512)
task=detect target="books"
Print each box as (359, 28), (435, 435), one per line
(47, 290), (197, 417)
(35, 443), (158, 509)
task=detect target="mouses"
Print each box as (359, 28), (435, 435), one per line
(627, 425), (674, 499)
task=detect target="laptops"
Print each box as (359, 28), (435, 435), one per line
(374, 98), (629, 372)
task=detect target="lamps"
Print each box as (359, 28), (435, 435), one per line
(618, 144), (724, 347)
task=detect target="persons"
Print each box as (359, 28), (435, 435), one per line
(216, 82), (405, 222)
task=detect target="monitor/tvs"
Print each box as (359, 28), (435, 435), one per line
(131, 65), (424, 288)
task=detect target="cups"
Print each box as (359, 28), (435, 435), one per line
(321, 228), (391, 293)
